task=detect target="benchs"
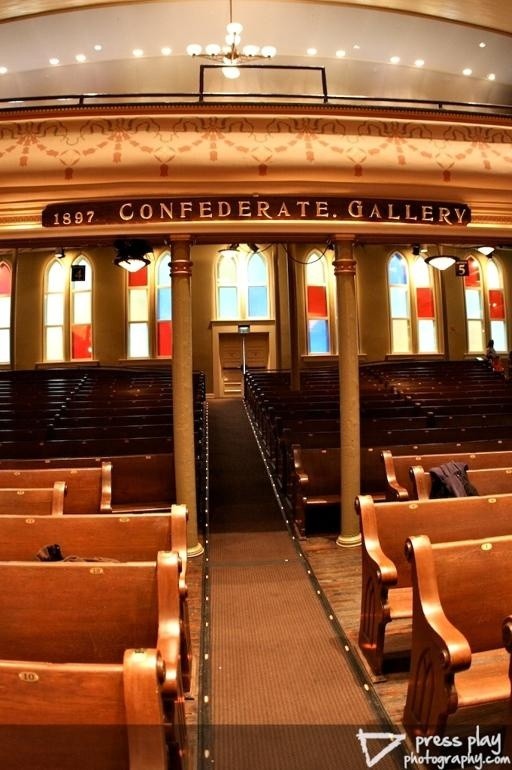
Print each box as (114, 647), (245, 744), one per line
(240, 359), (510, 770)
(1, 358), (216, 770)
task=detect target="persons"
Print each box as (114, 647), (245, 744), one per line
(486, 340), (496, 366)
